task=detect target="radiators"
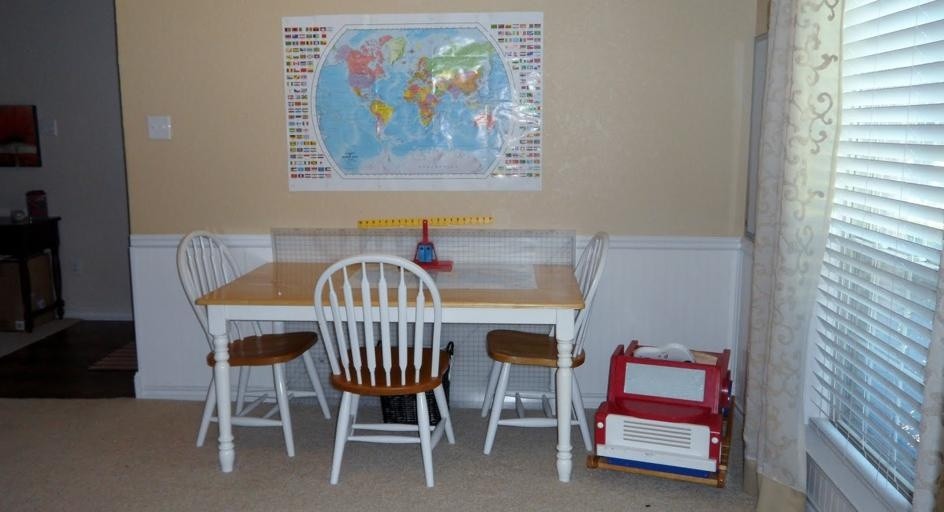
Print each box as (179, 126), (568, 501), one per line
(801, 419), (911, 512)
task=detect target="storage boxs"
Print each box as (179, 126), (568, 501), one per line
(0, 252), (56, 332)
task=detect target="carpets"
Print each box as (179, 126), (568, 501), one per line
(0, 319), (137, 400)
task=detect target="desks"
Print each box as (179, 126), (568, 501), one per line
(1, 217), (63, 334)
(195, 261), (586, 483)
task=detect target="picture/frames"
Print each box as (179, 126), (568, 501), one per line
(744, 32), (769, 240)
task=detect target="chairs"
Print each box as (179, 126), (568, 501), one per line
(483, 230), (610, 456)
(177, 230), (332, 458)
(313, 253), (457, 487)
